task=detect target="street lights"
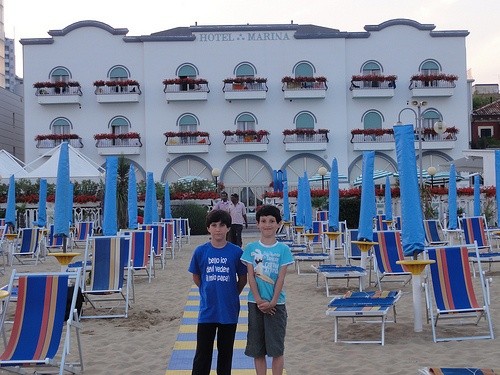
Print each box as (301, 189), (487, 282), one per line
(212, 168), (220, 193)
(428, 167), (437, 189)
(318, 167), (327, 190)
(395, 105), (423, 189)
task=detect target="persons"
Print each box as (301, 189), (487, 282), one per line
(211, 192), (263, 249)
(188, 210), (247, 375)
(240, 204), (295, 375)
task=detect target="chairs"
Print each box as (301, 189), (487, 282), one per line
(0, 217), (191, 375)
(275, 211), (500, 375)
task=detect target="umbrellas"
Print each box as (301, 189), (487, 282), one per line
(282, 124), (499, 260)
(5, 143), (171, 262)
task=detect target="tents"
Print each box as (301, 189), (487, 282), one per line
(0, 142), (102, 184)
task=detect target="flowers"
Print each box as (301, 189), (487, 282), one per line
(352, 73), (397, 81)
(222, 130), (269, 136)
(164, 131), (209, 137)
(163, 78), (208, 84)
(35, 133), (79, 141)
(351, 129), (394, 135)
(280, 76), (327, 82)
(282, 128), (330, 135)
(411, 74), (458, 81)
(223, 76), (267, 84)
(94, 132), (141, 140)
(93, 80), (140, 87)
(424, 126), (459, 135)
(32, 81), (80, 88)
